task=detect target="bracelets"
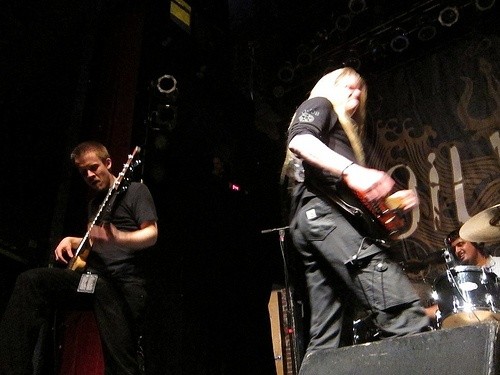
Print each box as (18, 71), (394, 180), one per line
(342, 162), (357, 175)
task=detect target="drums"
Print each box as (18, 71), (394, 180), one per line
(353, 311), (382, 344)
(432, 266), (500, 330)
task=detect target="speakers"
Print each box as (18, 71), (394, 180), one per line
(298, 321), (500, 375)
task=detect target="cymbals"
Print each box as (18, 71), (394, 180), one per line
(459, 204), (500, 243)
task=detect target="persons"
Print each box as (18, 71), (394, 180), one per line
(280, 67), (429, 375)
(0, 141), (159, 375)
(446, 230), (500, 276)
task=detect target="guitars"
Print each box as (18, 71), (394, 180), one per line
(68, 146), (141, 272)
